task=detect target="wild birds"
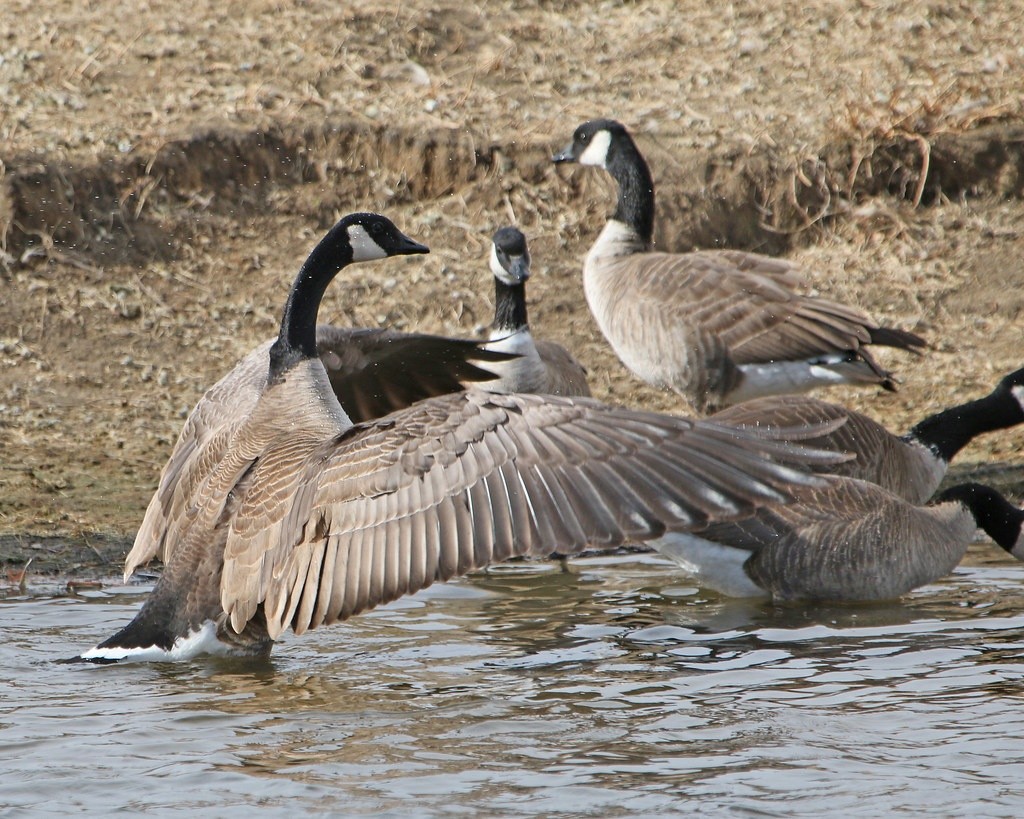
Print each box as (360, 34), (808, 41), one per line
(642, 473), (1024, 603)
(706, 366), (1024, 506)
(50, 212), (857, 664)
(460, 228), (592, 395)
(549, 118), (934, 419)
